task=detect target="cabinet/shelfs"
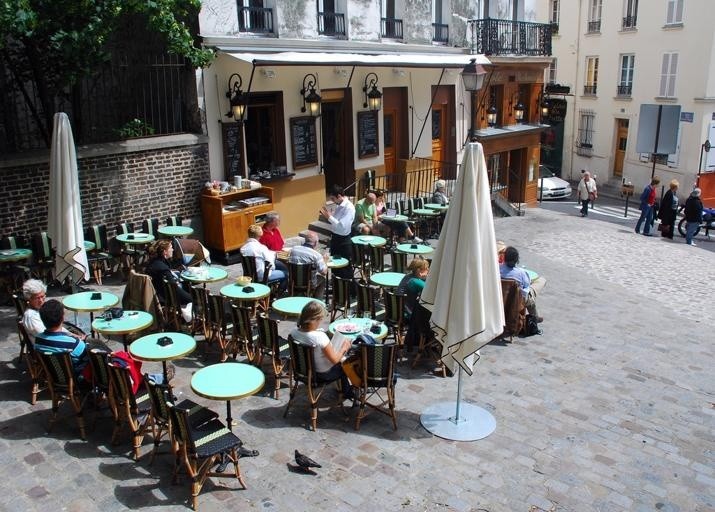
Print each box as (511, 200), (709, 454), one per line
(199, 185), (274, 266)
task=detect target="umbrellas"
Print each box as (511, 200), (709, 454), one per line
(47, 114), (92, 327)
(417, 142), (509, 423)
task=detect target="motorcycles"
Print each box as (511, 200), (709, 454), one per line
(677, 204), (715, 238)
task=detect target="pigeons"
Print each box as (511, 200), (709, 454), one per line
(295, 449), (321, 472)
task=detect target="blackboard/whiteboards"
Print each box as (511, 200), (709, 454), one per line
(290, 116), (319, 171)
(357, 110), (379, 159)
(221, 122), (246, 183)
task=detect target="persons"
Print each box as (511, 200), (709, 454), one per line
(397, 239), (544, 375)
(635, 176), (661, 236)
(432, 180), (448, 204)
(577, 172), (598, 217)
(353, 193), (391, 235)
(144, 236), (193, 324)
(240, 224), (289, 294)
(366, 190), (424, 244)
(22, 278), (86, 350)
(32, 299), (175, 392)
(286, 231), (329, 298)
(258, 211), (286, 250)
(289, 300), (355, 399)
(318, 185), (357, 300)
(658, 180), (680, 239)
(685, 188), (704, 245)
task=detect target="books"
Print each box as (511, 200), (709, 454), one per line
(329, 331), (353, 355)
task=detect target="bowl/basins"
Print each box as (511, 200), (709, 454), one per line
(236, 276), (252, 285)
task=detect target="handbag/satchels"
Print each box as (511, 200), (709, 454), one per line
(588, 192), (596, 201)
(657, 224), (670, 232)
(519, 313), (538, 338)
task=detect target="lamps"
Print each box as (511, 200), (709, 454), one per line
(298, 74), (322, 119)
(480, 91), (553, 129)
(360, 72), (383, 117)
(221, 74), (249, 122)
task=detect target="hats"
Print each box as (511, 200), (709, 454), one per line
(437, 180), (445, 189)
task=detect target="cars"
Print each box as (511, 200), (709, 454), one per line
(537, 164), (572, 200)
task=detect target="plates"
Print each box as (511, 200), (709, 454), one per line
(334, 324), (361, 334)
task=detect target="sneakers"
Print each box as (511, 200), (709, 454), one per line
(412, 237), (424, 243)
(535, 316), (543, 322)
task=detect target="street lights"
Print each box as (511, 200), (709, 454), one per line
(461, 60), (485, 141)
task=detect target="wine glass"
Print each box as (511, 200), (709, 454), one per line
(364, 310), (371, 327)
(105, 312), (113, 328)
(346, 309), (354, 324)
